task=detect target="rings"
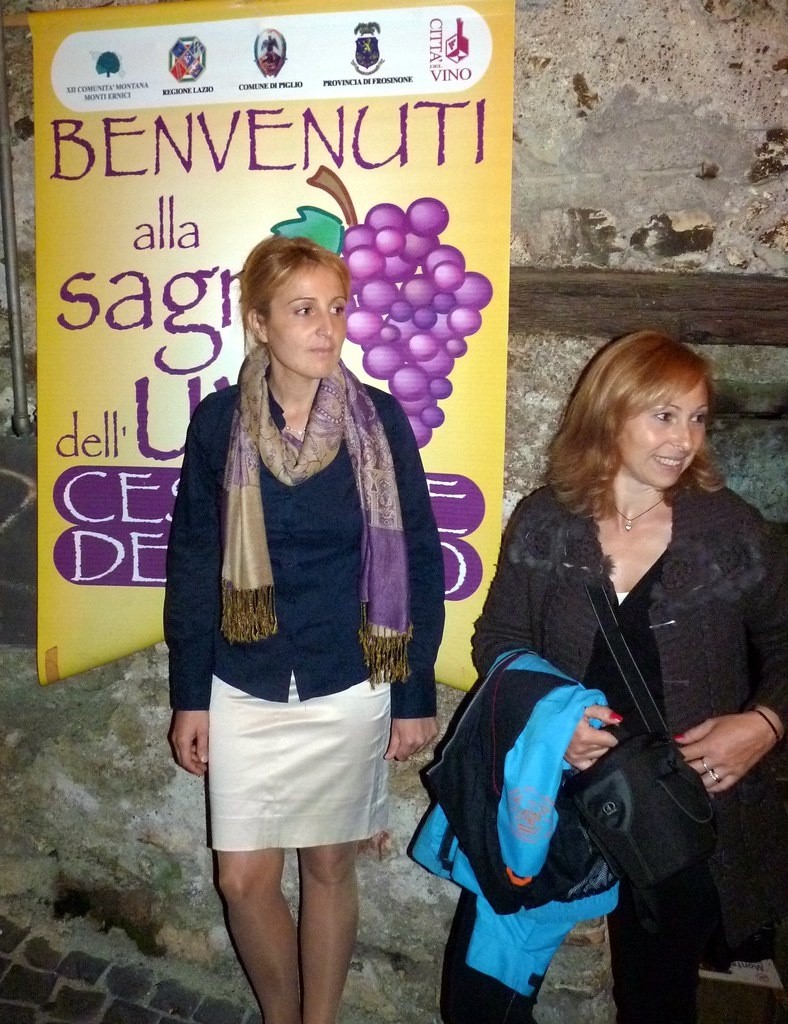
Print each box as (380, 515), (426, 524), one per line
(700, 758), (709, 772)
(708, 769), (722, 784)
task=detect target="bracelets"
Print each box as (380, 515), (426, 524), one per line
(750, 710), (780, 745)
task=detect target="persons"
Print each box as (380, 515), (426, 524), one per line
(441, 330), (788, 1024)
(163, 237), (446, 1024)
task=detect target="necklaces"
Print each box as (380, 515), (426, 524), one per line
(284, 426), (307, 443)
(614, 498), (664, 532)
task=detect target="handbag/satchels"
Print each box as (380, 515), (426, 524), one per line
(561, 733), (714, 889)
